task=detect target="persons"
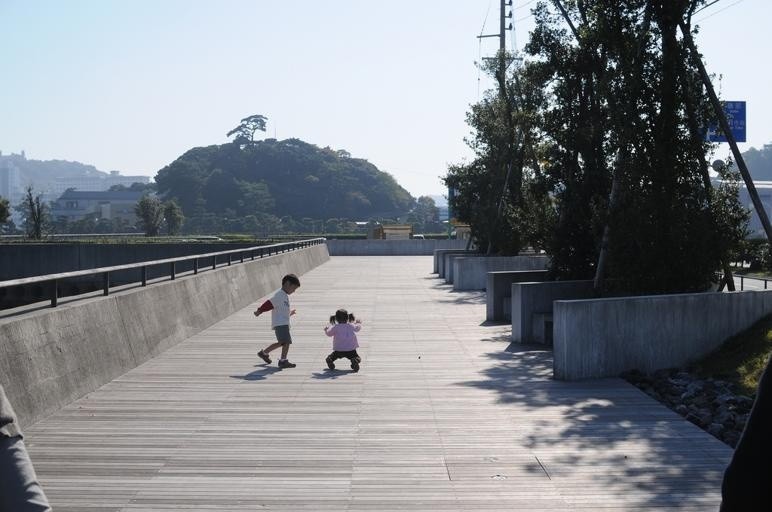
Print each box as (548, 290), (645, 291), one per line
(323, 309), (363, 371)
(253, 274), (301, 368)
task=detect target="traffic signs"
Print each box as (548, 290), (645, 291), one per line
(696, 101), (746, 142)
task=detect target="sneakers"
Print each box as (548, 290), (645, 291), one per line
(257, 351), (272, 364)
(278, 360), (296, 368)
(326, 357), (335, 369)
(351, 361), (359, 372)
